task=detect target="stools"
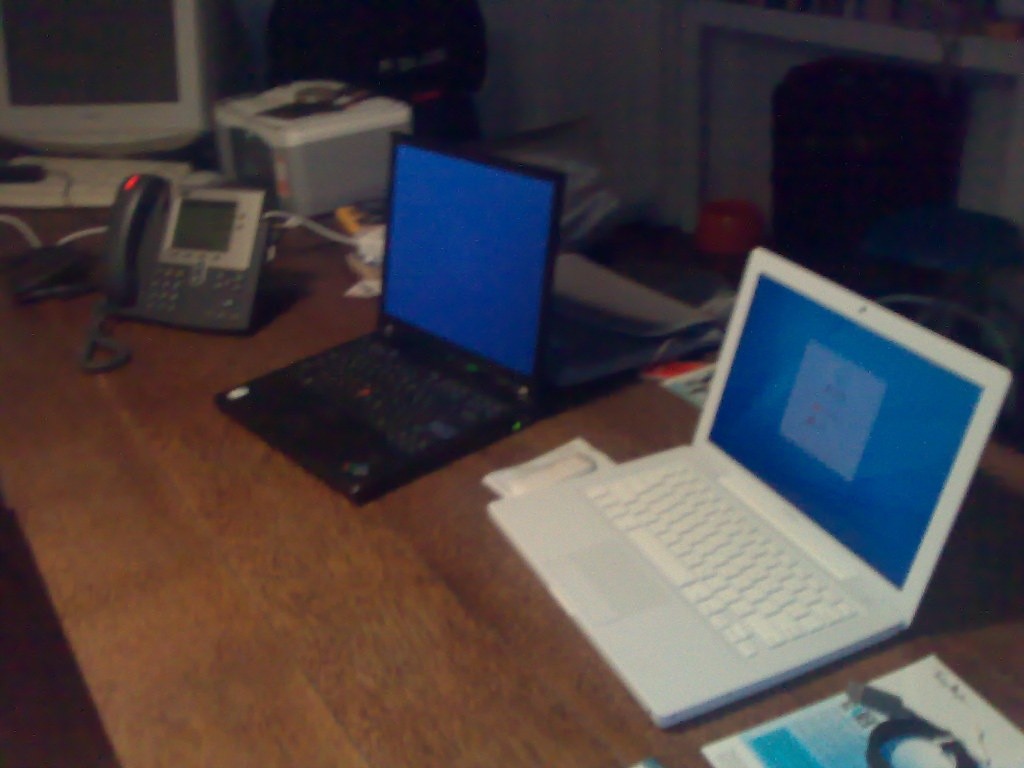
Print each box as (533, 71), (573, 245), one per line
(859, 205), (1021, 426)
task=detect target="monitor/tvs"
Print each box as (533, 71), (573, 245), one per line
(0, 0), (219, 160)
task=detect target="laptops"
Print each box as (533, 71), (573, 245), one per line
(215, 129), (564, 505)
(492, 247), (1014, 728)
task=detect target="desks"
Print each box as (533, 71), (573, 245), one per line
(0, 200), (1024, 768)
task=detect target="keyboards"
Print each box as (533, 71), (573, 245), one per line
(0, 155), (189, 210)
